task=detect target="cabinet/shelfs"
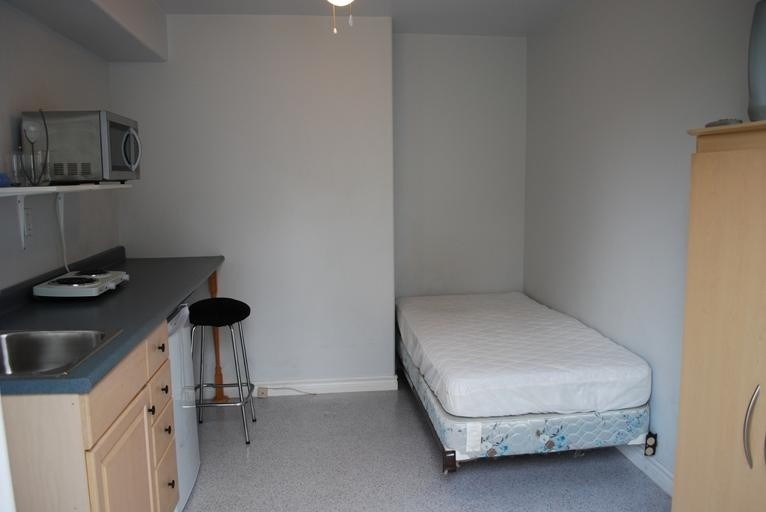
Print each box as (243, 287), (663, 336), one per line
(668, 117), (764, 512)
(3, 319), (181, 512)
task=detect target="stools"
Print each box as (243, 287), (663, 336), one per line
(187, 297), (256, 444)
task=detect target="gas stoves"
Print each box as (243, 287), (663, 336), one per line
(32, 269), (131, 298)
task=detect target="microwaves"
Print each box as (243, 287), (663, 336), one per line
(19, 110), (142, 185)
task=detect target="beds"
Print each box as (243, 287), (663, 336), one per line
(395, 292), (656, 476)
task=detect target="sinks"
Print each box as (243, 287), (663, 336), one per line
(0, 328), (104, 375)
